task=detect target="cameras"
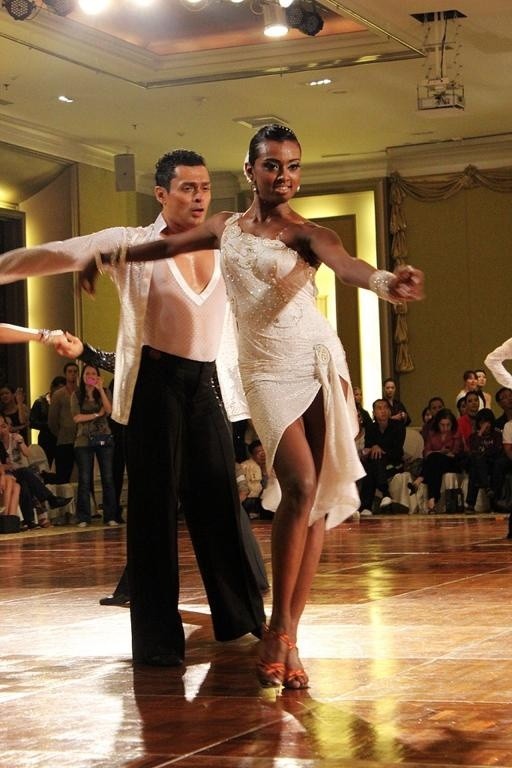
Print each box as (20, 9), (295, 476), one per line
(87, 376), (97, 386)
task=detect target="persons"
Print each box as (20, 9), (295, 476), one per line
(1, 149), (270, 668)
(344, 371), (511, 539)
(80, 124), (424, 688)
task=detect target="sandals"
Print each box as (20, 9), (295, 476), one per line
(254, 622), (291, 690)
(283, 642), (311, 690)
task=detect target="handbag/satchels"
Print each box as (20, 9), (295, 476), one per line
(2, 516), (21, 533)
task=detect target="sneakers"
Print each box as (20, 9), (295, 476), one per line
(26, 519), (117, 527)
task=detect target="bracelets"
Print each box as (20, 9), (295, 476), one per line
(369, 269), (402, 304)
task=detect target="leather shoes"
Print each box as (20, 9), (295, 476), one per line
(51, 496), (73, 510)
(100, 594), (131, 606)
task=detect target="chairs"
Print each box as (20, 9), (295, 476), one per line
(29, 444), (60, 517)
(389, 427), (487, 511)
